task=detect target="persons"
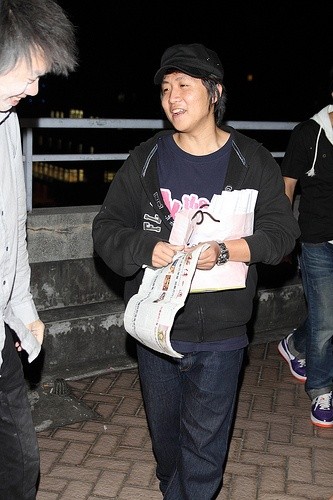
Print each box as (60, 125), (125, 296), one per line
(0, 0), (79, 500)
(277, 83), (333, 428)
(91, 45), (301, 500)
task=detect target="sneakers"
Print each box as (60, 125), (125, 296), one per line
(278, 334), (308, 380)
(310, 393), (333, 427)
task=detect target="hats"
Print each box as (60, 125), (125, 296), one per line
(154, 43), (224, 86)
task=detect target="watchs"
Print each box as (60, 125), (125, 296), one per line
(216, 240), (229, 266)
